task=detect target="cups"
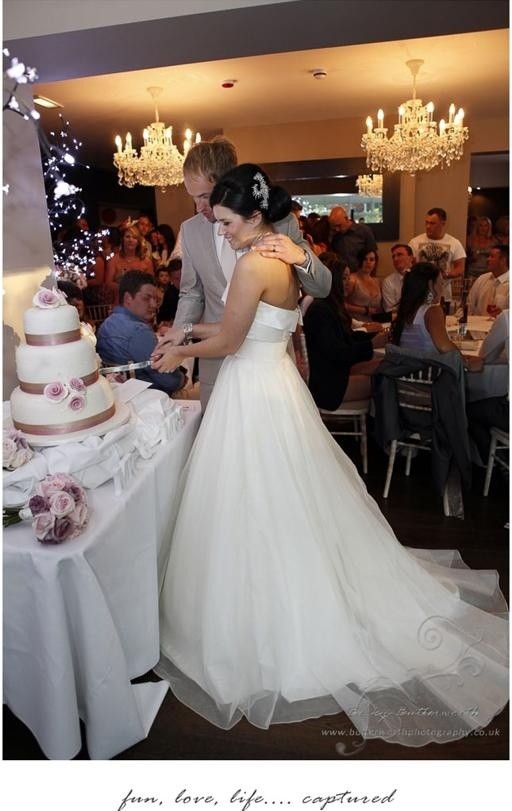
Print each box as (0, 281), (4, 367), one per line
(457, 324), (467, 336)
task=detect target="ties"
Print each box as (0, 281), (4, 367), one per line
(222, 236), (237, 281)
(488, 279), (499, 305)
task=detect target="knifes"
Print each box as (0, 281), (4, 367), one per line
(96, 361), (153, 374)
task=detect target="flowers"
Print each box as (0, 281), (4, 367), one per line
(16, 469), (89, 545)
(3, 428), (33, 473)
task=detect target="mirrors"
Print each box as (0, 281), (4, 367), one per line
(251, 155), (399, 241)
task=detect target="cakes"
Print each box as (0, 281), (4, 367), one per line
(10, 289), (114, 435)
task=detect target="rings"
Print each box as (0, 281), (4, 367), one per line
(273, 244), (275, 252)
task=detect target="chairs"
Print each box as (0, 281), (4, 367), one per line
(285, 301), (369, 476)
(483, 427), (509, 533)
(373, 353), (470, 517)
(441, 277), (477, 301)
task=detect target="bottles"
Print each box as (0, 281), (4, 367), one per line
(456, 291), (467, 323)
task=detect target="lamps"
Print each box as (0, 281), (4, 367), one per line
(114, 87), (202, 193)
(353, 169), (382, 198)
(362, 55), (468, 173)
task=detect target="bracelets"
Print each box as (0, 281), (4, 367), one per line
(182, 323), (193, 341)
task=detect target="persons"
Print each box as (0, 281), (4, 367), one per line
(301, 253), (374, 411)
(66, 213), (200, 399)
(477, 309), (508, 364)
(148, 162), (508, 746)
(388, 262), (466, 368)
(148, 138), (334, 418)
(290, 199), (509, 321)
